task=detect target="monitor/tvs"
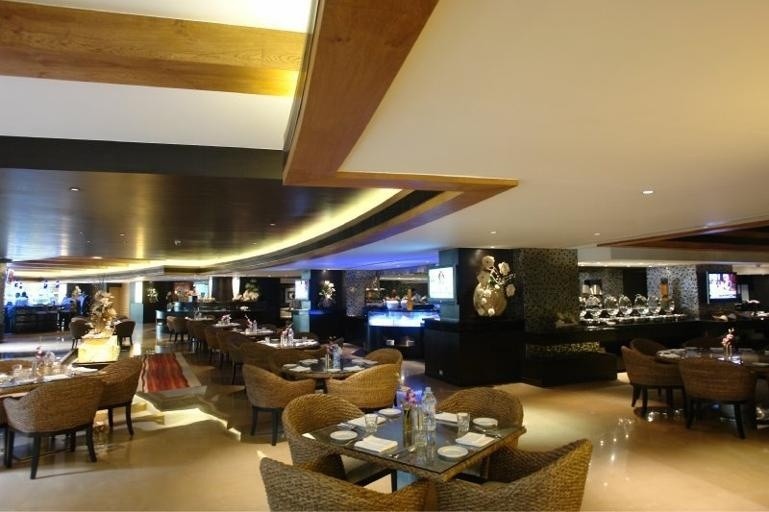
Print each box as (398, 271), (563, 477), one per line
(705, 270), (737, 305)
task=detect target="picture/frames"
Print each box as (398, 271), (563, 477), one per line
(284, 287), (295, 304)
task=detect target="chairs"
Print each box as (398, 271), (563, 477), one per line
(91, 355), (143, 435)
(620, 345), (682, 422)
(679, 357), (758, 439)
(71, 313), (135, 350)
(0, 359), (33, 372)
(2, 376), (104, 480)
(630, 339), (666, 401)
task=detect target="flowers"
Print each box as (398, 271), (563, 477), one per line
(477, 254), (518, 318)
(242, 282), (260, 301)
(90, 290), (118, 334)
(317, 279), (336, 301)
(720, 327), (735, 347)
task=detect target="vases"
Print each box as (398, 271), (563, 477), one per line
(723, 345), (732, 357)
(473, 285), (507, 318)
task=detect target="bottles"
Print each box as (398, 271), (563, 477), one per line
(419, 386), (438, 432)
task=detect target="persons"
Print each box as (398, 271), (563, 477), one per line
(188, 286), (197, 296)
(402, 288), (422, 302)
(5, 288), (90, 332)
(390, 287), (400, 300)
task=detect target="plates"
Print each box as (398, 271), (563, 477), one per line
(580, 292), (677, 321)
(330, 406), (500, 459)
(284, 359), (373, 375)
